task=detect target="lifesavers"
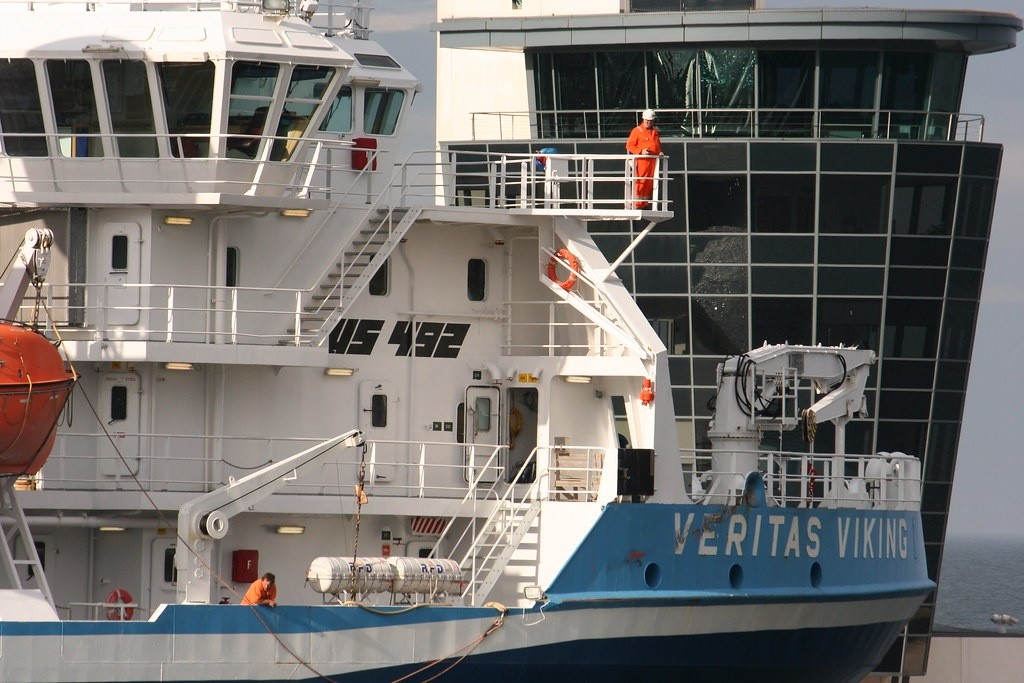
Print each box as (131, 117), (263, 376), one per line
(105, 588), (135, 620)
(510, 408), (523, 435)
(547, 248), (580, 292)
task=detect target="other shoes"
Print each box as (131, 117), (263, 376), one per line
(642, 205), (651, 210)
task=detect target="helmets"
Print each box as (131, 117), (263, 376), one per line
(642, 109), (655, 120)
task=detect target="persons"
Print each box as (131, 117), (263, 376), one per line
(625, 109), (665, 210)
(238, 572), (277, 608)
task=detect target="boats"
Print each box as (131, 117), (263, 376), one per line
(0, 1), (942, 682)
